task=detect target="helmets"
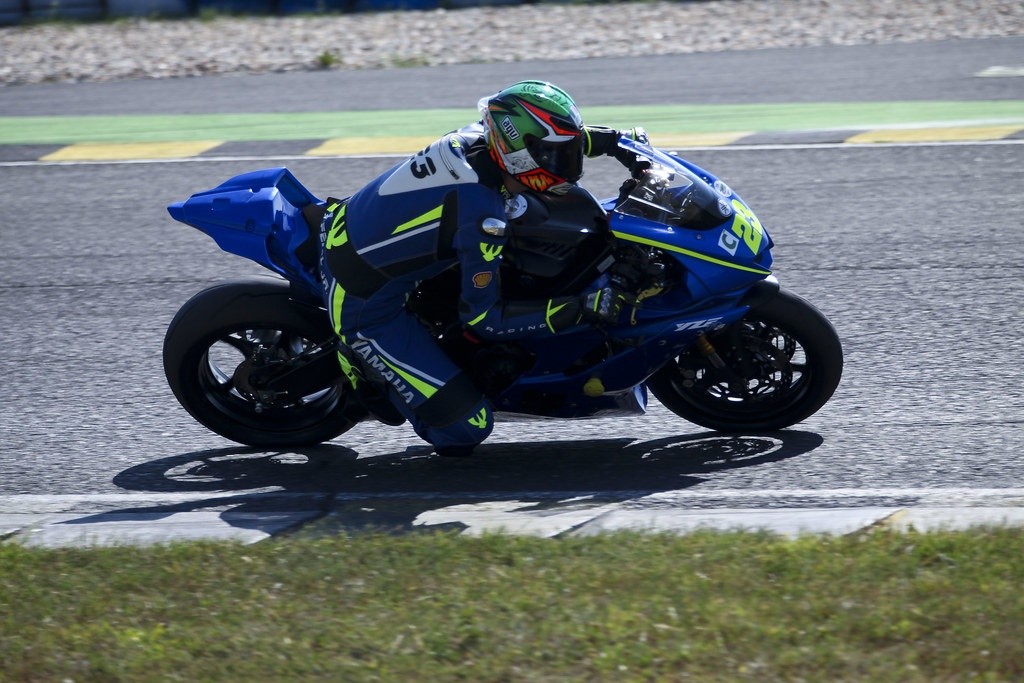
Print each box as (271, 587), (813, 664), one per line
(477, 80), (584, 195)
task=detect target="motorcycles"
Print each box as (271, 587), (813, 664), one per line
(164, 124), (843, 454)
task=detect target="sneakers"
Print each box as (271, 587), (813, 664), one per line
(338, 346), (407, 426)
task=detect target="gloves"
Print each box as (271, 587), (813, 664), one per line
(545, 286), (626, 334)
(584, 125), (654, 170)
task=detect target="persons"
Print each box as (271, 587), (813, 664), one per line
(320, 79), (651, 457)
